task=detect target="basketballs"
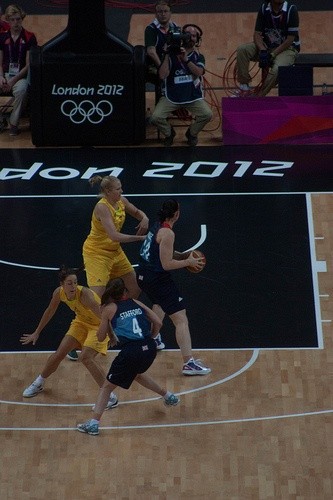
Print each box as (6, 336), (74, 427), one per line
(184, 250), (206, 273)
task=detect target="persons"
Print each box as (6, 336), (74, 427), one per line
(0, 0), (301, 146)
(136, 199), (211, 376)
(77, 277), (179, 435)
(66, 177), (149, 359)
(20, 270), (118, 411)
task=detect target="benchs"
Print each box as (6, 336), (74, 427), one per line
(261, 53), (333, 85)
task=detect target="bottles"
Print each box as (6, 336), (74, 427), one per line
(321, 82), (328, 95)
(146, 107), (152, 136)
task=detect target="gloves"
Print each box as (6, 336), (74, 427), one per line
(257, 49), (274, 68)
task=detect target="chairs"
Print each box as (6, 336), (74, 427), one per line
(154, 81), (193, 140)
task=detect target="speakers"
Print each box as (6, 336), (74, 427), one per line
(278, 65), (314, 97)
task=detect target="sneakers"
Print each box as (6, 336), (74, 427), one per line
(91, 396), (119, 411)
(183, 359), (211, 375)
(153, 333), (165, 350)
(23, 380), (46, 397)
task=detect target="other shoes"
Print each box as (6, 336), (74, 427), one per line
(160, 393), (179, 408)
(0, 119), (7, 132)
(66, 349), (79, 360)
(78, 422), (99, 435)
(185, 128), (197, 146)
(230, 89), (251, 97)
(164, 128), (176, 145)
(8, 122), (18, 136)
